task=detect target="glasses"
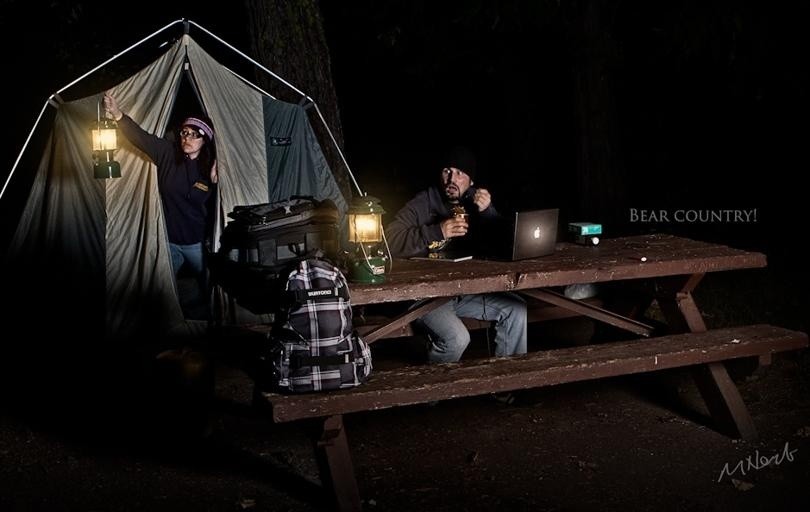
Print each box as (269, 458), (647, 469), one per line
(180, 130), (201, 138)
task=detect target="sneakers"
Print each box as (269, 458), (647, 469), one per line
(495, 396), (541, 413)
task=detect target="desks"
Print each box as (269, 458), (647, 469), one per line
(206, 230), (768, 511)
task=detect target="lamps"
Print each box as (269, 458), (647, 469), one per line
(344, 193), (389, 284)
(88, 110), (122, 179)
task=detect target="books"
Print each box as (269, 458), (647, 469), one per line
(409, 245), (473, 262)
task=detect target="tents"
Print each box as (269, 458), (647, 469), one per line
(9, 34), (367, 374)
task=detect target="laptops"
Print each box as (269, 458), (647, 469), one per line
(474, 208), (560, 262)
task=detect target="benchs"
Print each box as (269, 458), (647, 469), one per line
(253, 324), (809, 512)
(345, 299), (651, 346)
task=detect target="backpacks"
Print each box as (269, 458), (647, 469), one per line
(270, 255), (373, 393)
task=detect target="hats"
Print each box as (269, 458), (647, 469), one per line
(445, 144), (482, 183)
(183, 113), (213, 140)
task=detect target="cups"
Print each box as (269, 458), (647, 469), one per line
(452, 213), (470, 229)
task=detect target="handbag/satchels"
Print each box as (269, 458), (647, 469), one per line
(226, 195), (341, 240)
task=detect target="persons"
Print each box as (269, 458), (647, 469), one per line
(378, 154), (538, 411)
(103, 93), (219, 277)
